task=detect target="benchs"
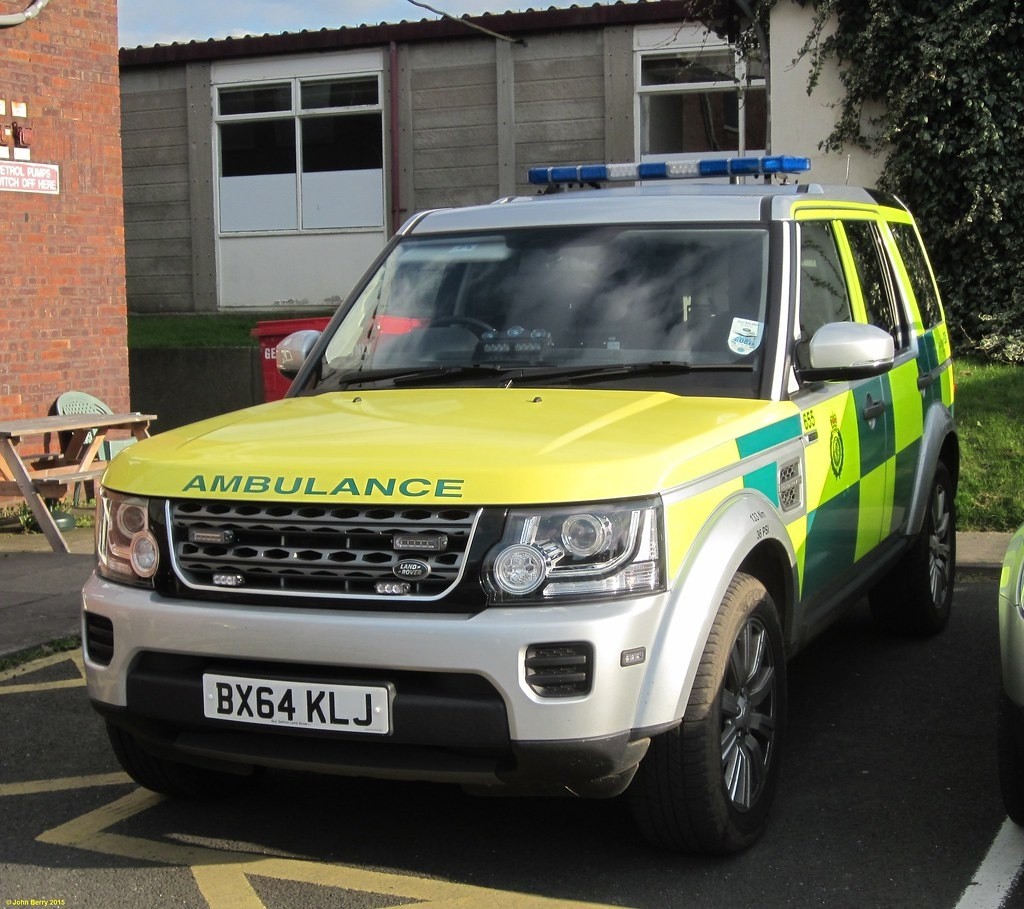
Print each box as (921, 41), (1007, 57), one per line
(17, 451), (63, 464)
(35, 470), (109, 485)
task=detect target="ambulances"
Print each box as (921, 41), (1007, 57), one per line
(80, 155), (958, 858)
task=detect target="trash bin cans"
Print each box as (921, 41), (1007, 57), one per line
(247, 317), (422, 403)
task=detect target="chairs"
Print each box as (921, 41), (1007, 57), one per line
(56, 391), (142, 460)
(663, 255), (762, 367)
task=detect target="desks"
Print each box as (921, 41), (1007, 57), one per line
(0, 410), (158, 551)
